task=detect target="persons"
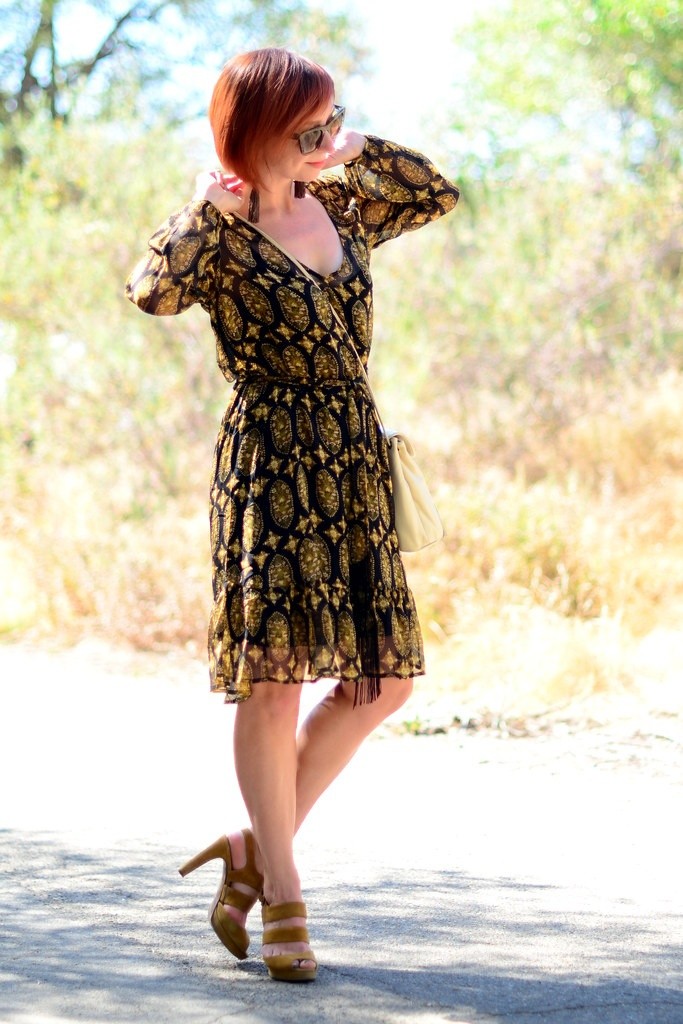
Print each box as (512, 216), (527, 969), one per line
(125, 48), (459, 982)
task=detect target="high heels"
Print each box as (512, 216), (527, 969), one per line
(178, 829), (264, 959)
(258, 887), (319, 981)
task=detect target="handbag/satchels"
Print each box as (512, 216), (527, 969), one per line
(386, 430), (447, 553)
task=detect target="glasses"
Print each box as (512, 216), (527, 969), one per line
(282, 104), (346, 155)
